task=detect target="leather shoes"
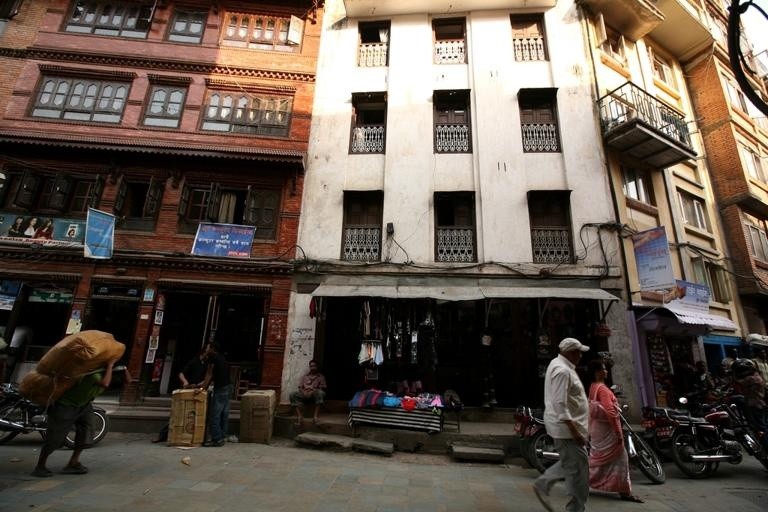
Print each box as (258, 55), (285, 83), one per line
(202, 440), (225, 447)
(152, 435), (160, 443)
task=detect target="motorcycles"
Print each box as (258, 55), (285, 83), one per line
(0, 381), (108, 450)
(512, 383), (766, 482)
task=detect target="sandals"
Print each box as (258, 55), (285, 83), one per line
(620, 494), (645, 503)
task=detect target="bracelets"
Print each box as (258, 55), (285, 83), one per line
(199, 387), (204, 391)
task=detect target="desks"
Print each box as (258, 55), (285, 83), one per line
(349, 405), (442, 438)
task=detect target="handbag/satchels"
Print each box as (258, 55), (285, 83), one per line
(588, 398), (607, 420)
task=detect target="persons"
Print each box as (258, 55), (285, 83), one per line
(30, 359), (115, 478)
(532, 337), (590, 512)
(0, 217), (54, 260)
(583, 362), (644, 503)
(289, 361), (328, 428)
(684, 347), (768, 440)
(194, 342), (231, 447)
(149, 346), (207, 443)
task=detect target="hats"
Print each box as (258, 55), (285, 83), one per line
(558, 337), (590, 353)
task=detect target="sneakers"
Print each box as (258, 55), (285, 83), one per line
(65, 462), (86, 470)
(33, 466), (53, 478)
(532, 482), (555, 512)
